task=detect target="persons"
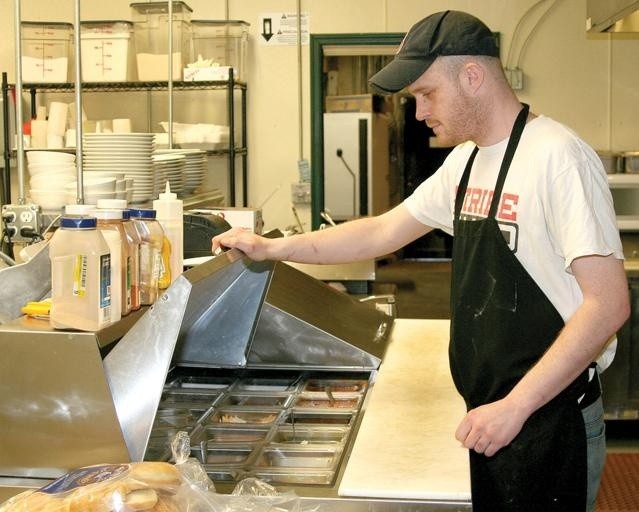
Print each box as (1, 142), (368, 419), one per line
(373, 95), (399, 198)
(211, 11), (631, 511)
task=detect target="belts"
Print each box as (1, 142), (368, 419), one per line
(579, 369), (603, 410)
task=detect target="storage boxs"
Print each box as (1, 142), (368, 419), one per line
(17, 0), (251, 85)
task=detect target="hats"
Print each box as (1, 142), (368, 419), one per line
(367, 8), (500, 95)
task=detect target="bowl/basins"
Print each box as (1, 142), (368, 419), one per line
(24, 150), (116, 212)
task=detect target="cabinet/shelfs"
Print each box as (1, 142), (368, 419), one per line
(18, 67), (254, 208)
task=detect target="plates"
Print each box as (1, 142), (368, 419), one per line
(80, 134), (208, 205)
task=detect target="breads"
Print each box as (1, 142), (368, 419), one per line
(0, 462), (188, 511)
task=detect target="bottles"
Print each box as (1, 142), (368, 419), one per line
(48, 182), (184, 331)
